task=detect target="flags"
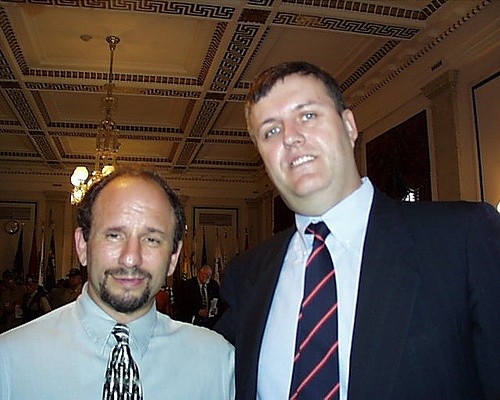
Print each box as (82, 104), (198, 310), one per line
(212, 231), (222, 287)
(235, 234), (240, 255)
(222, 232), (228, 269)
(44, 229), (57, 290)
(201, 232), (207, 265)
(182, 234), (190, 280)
(12, 230), (25, 283)
(191, 233), (198, 278)
(172, 253), (179, 280)
(38, 229), (45, 288)
(244, 232), (249, 252)
(27, 227), (37, 281)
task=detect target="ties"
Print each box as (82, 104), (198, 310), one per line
(287, 220), (341, 400)
(102, 323), (144, 400)
(201, 284), (207, 309)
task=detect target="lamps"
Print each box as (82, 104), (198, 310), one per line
(70, 36), (120, 206)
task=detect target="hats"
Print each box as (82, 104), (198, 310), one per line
(67, 268), (80, 277)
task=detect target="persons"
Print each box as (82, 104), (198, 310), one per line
(46, 268), (83, 310)
(0, 166), (235, 400)
(212, 61), (500, 400)
(174, 265), (222, 330)
(22, 275), (51, 311)
